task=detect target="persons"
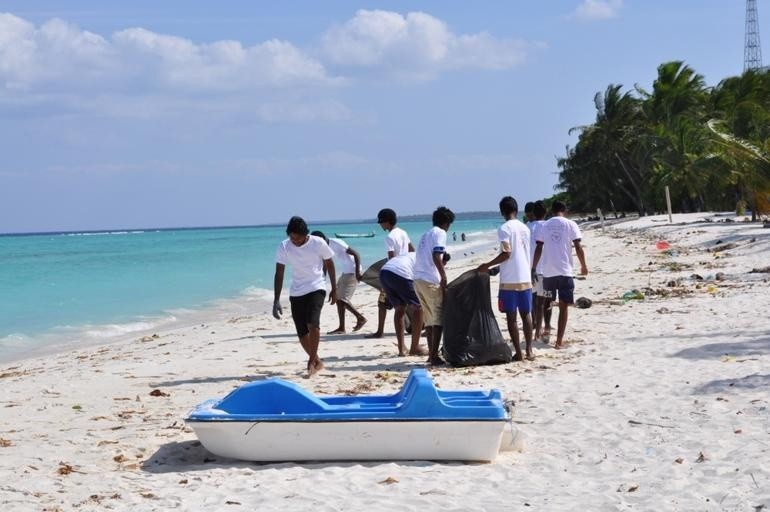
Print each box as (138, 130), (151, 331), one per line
(273, 215), (339, 375)
(529, 201), (553, 339)
(379, 249), (451, 356)
(452, 231), (457, 242)
(531, 201), (588, 350)
(523, 202), (556, 331)
(311, 230), (367, 335)
(412, 206), (456, 366)
(363, 208), (416, 339)
(477, 196), (535, 361)
(461, 232), (466, 240)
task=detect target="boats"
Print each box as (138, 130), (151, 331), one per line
(183, 367), (521, 464)
(336, 230), (376, 239)
